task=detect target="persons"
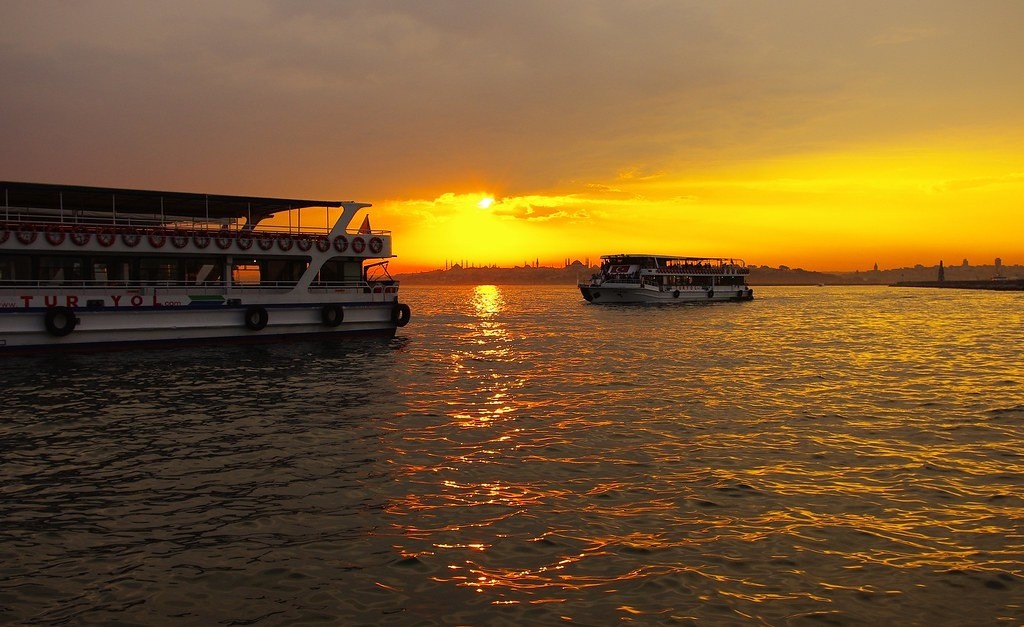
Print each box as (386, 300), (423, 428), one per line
(589, 259), (711, 285)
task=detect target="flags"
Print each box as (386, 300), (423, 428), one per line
(359, 216), (370, 234)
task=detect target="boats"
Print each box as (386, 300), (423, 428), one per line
(576, 253), (754, 304)
(0, 180), (411, 357)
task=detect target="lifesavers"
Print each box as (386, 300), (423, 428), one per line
(70, 224), (90, 245)
(258, 234), (273, 250)
(45, 306), (77, 337)
(278, 234), (383, 254)
(193, 231), (211, 248)
(215, 231), (233, 249)
(237, 233), (253, 250)
(97, 228), (116, 247)
(16, 223), (37, 245)
(148, 230), (166, 247)
(659, 269), (750, 274)
(46, 223), (65, 245)
(172, 229), (189, 248)
(391, 303), (412, 327)
(245, 306), (269, 329)
(673, 289), (753, 298)
(122, 227), (140, 247)
(0, 224), (9, 244)
(319, 303), (345, 328)
